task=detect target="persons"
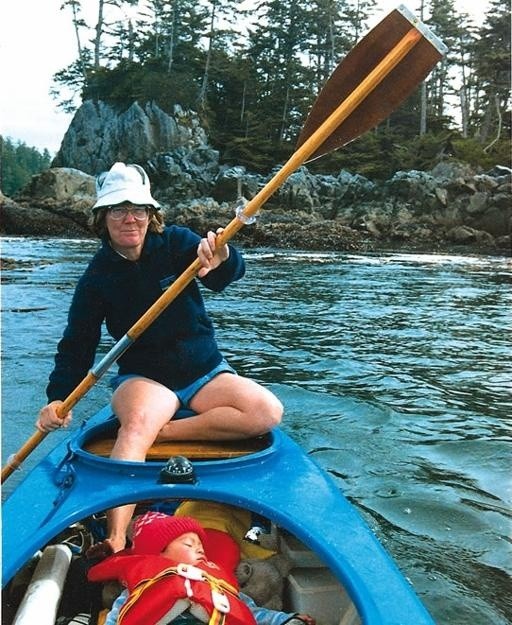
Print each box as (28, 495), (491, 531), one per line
(34, 159), (285, 561)
(105, 509), (317, 625)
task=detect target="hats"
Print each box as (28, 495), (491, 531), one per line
(91, 160), (162, 210)
(130, 509), (210, 556)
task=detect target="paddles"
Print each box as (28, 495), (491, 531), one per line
(0, 5), (448, 487)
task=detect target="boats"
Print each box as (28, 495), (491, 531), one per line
(1, 401), (436, 625)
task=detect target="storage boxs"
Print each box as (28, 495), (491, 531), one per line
(286, 571), (350, 625)
(277, 533), (328, 567)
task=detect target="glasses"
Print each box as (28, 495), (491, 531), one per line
(105, 204), (149, 220)
